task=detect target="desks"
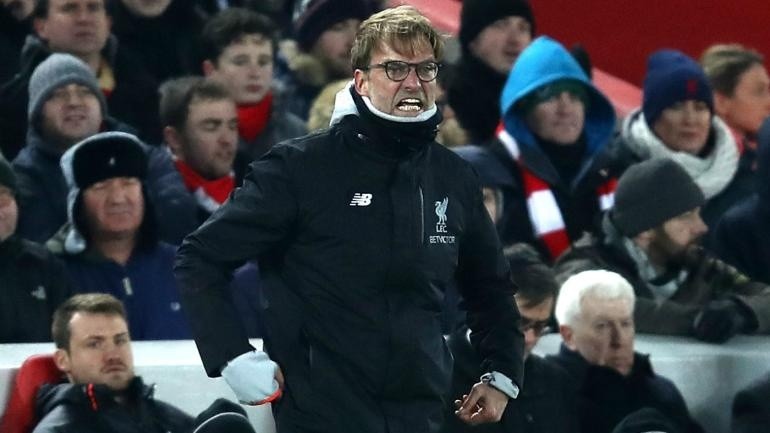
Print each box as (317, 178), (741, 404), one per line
(0, 341), (278, 433)
(531, 327), (769, 433)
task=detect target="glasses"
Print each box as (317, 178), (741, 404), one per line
(368, 60), (442, 81)
(518, 313), (553, 336)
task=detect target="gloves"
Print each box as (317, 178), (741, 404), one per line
(688, 298), (747, 345)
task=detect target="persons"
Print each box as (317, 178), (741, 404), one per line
(173, 6), (525, 433)
(545, 269), (706, 432)
(0, 0), (770, 376)
(33, 293), (256, 433)
(446, 244), (576, 433)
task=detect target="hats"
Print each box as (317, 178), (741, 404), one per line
(292, 0), (381, 55)
(27, 53), (107, 127)
(458, 0), (537, 47)
(611, 159), (704, 236)
(59, 132), (159, 255)
(641, 52), (715, 128)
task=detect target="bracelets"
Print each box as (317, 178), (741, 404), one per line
(479, 371), (519, 399)
